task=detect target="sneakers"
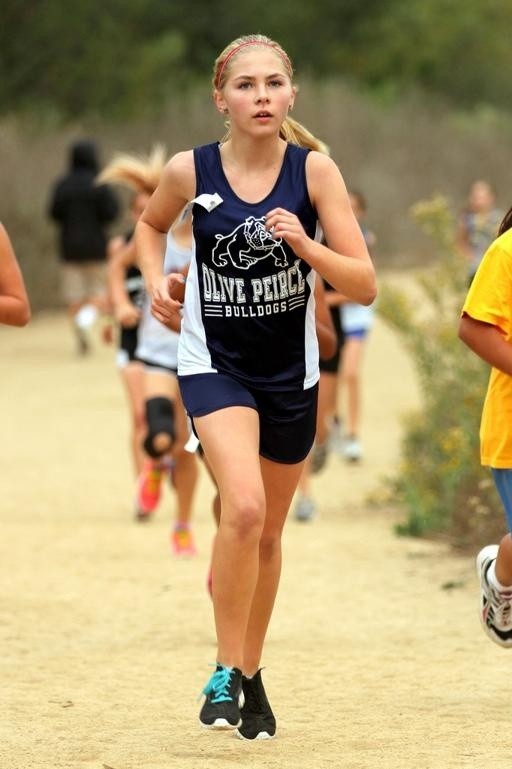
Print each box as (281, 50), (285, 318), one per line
(199, 663), (243, 732)
(476, 544), (512, 649)
(236, 667), (277, 743)
(129, 416), (366, 557)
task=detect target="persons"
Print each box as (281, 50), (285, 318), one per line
(132, 32), (380, 745)
(294, 192), (380, 520)
(455, 208), (511, 651)
(45, 133), (124, 353)
(98, 142), (222, 595)
(451, 178), (509, 290)
(0, 221), (34, 336)
